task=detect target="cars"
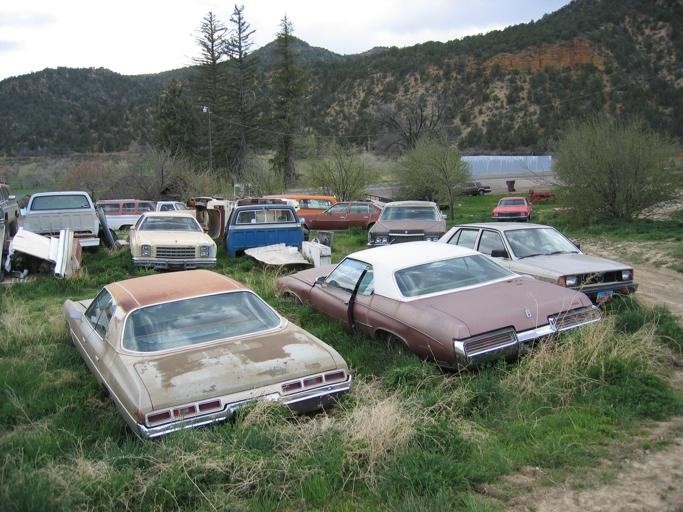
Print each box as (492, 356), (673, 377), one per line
(367, 200), (449, 247)
(276, 240), (604, 373)
(461, 182), (492, 195)
(490, 196), (534, 222)
(63, 266), (353, 443)
(438, 222), (641, 304)
